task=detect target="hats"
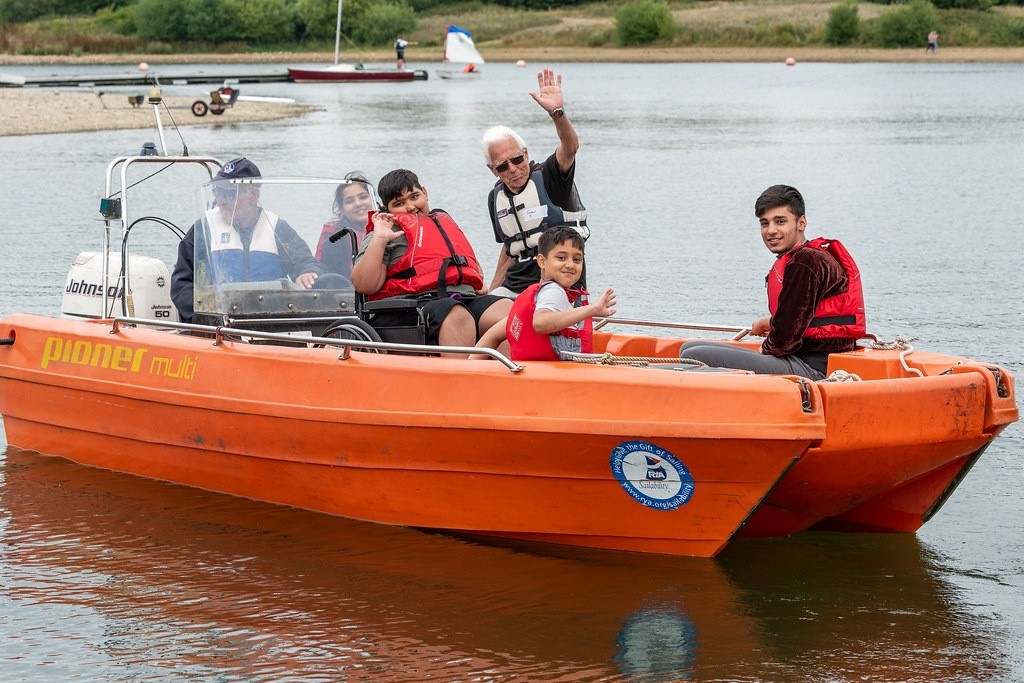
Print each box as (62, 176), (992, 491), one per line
(209, 158), (262, 190)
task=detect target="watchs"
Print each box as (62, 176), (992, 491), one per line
(549, 108), (565, 118)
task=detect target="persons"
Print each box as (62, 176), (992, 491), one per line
(679, 184), (866, 378)
(467, 226), (617, 361)
(926, 31), (939, 54)
(315, 171), (374, 275)
(483, 66), (591, 296)
(351, 169), (514, 361)
(394, 36), (418, 70)
(170, 158), (329, 325)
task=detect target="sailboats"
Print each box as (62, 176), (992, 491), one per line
(284, 0), (429, 82)
(435, 25), (487, 79)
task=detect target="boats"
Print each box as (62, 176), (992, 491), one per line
(0, 88), (1019, 562)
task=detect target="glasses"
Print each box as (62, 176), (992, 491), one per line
(491, 152), (524, 172)
(212, 187), (254, 199)
